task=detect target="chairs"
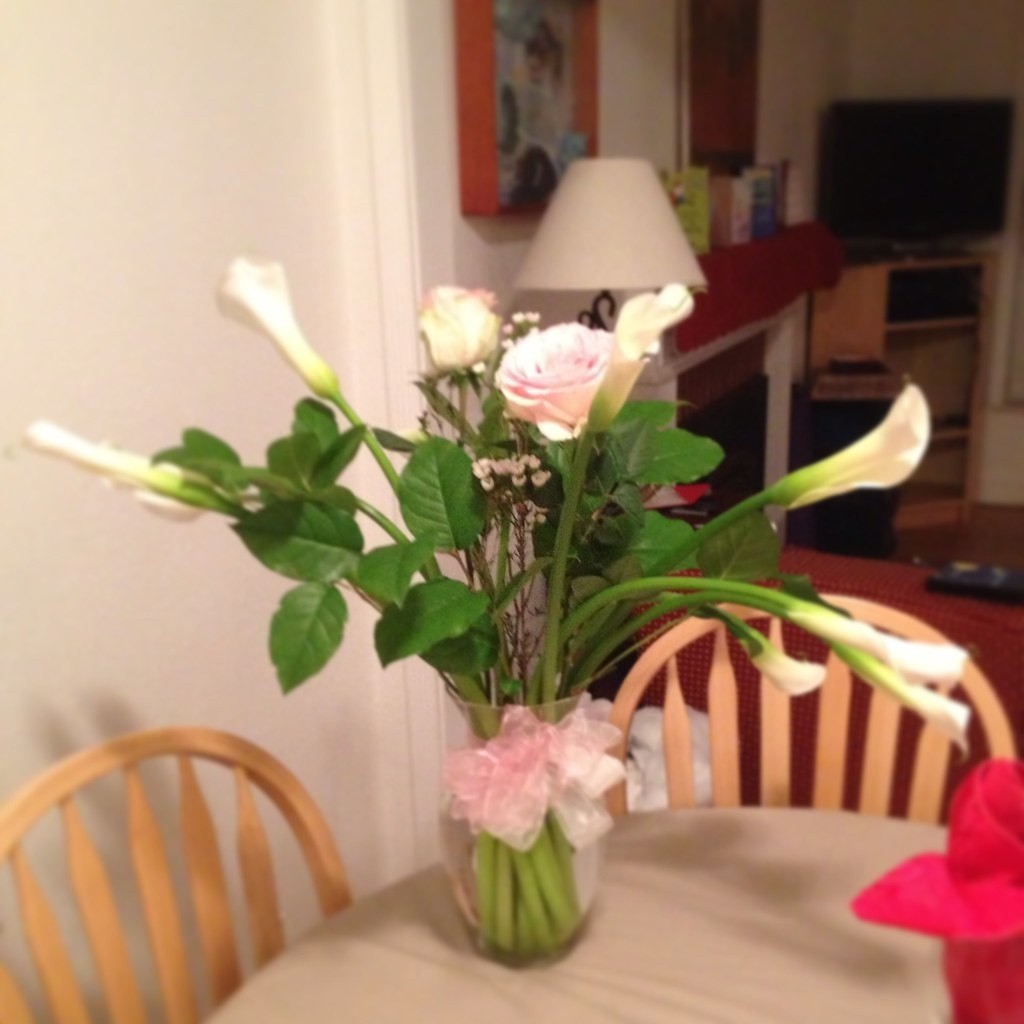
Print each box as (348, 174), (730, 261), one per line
(2, 724), (359, 1023)
(599, 593), (1020, 833)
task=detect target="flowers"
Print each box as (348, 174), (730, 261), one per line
(22, 245), (972, 959)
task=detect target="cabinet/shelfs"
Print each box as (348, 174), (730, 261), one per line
(808, 256), (999, 532)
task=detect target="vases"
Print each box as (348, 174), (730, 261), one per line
(438, 685), (604, 966)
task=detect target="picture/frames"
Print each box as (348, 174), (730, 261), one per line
(679, 3), (763, 177)
(453, 0), (600, 217)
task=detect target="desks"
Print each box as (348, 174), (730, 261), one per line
(194, 808), (957, 1024)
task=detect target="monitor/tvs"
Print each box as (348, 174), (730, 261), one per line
(821, 100), (1005, 236)
